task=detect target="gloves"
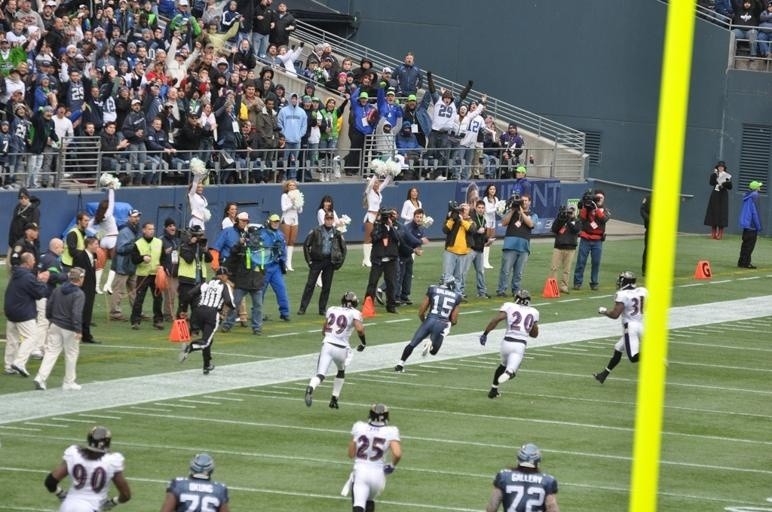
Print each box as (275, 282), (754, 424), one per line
(103, 498), (116, 510)
(57, 488), (66, 500)
(384, 463), (394, 473)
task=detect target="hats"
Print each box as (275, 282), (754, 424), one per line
(568, 205), (576, 211)
(749, 181), (762, 190)
(715, 161), (727, 169)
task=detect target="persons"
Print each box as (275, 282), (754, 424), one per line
(161, 452), (229, 512)
(705, 161), (731, 239)
(596, 269), (648, 384)
(738, 176), (766, 270)
(46, 425), (131, 512)
(574, 189), (608, 290)
(1, 2), (530, 180)
(638, 192), (653, 273)
(32, 268), (87, 391)
(551, 207), (582, 294)
(6, 255), (48, 377)
(4, 184), (504, 347)
(306, 290), (367, 409)
(394, 273), (462, 373)
(480, 289), (540, 401)
(496, 195), (537, 294)
(697, 0), (771, 68)
(483, 445), (563, 512)
(341, 401), (403, 511)
(179, 266), (237, 376)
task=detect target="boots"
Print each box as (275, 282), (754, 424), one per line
(95, 269), (103, 295)
(315, 269), (323, 288)
(483, 246), (493, 268)
(712, 226), (717, 239)
(103, 270), (116, 294)
(362, 244), (373, 267)
(717, 227), (723, 239)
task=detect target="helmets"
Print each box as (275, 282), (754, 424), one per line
(190, 454), (214, 473)
(87, 427), (111, 450)
(368, 404), (389, 426)
(616, 271), (636, 289)
(439, 272), (455, 287)
(518, 444), (541, 469)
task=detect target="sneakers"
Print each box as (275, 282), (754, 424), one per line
(742, 264), (756, 269)
